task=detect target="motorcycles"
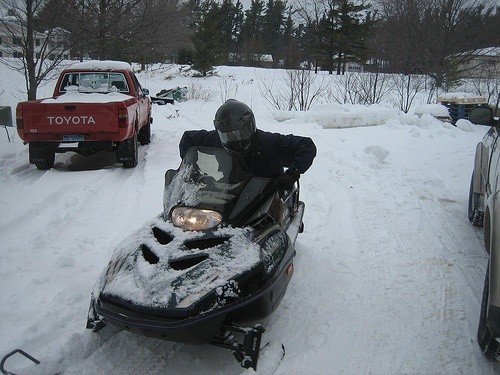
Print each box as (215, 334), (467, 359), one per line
(1, 147), (304, 375)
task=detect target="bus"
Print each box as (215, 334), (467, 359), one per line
(437, 46), (499, 128)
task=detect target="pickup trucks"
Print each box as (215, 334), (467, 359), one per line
(15, 60), (153, 168)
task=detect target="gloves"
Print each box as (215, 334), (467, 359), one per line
(184, 162), (202, 179)
(280, 167), (300, 193)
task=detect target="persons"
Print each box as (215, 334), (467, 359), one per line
(179, 98), (317, 228)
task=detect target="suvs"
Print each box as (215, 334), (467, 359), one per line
(468, 115), (500, 360)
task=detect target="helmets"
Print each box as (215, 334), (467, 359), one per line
(214, 100), (256, 152)
